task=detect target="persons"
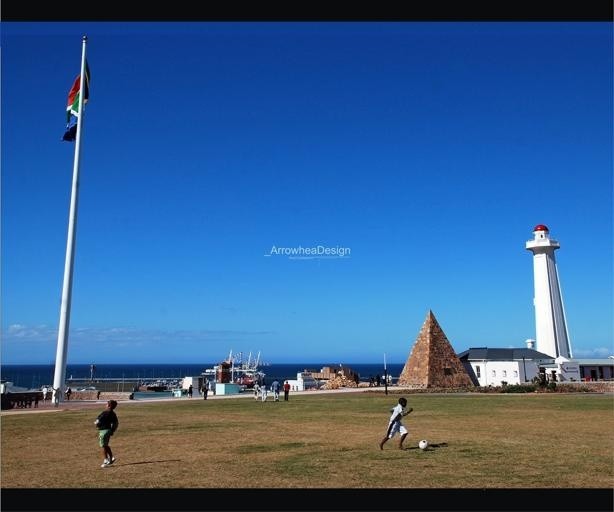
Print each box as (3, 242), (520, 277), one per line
(379, 398), (413, 450)
(202, 386), (209, 400)
(272, 379), (281, 401)
(97, 400), (119, 468)
(65, 387), (72, 400)
(370, 371), (393, 386)
(260, 383), (267, 402)
(253, 381), (261, 401)
(283, 382), (290, 401)
(189, 385), (193, 397)
(42, 386), (48, 401)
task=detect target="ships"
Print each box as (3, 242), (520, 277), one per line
(200, 348), (266, 388)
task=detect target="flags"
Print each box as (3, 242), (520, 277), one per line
(60, 59), (90, 142)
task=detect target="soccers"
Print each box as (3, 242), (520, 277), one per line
(418, 440), (429, 449)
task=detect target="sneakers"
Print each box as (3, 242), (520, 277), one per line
(100, 457), (116, 468)
